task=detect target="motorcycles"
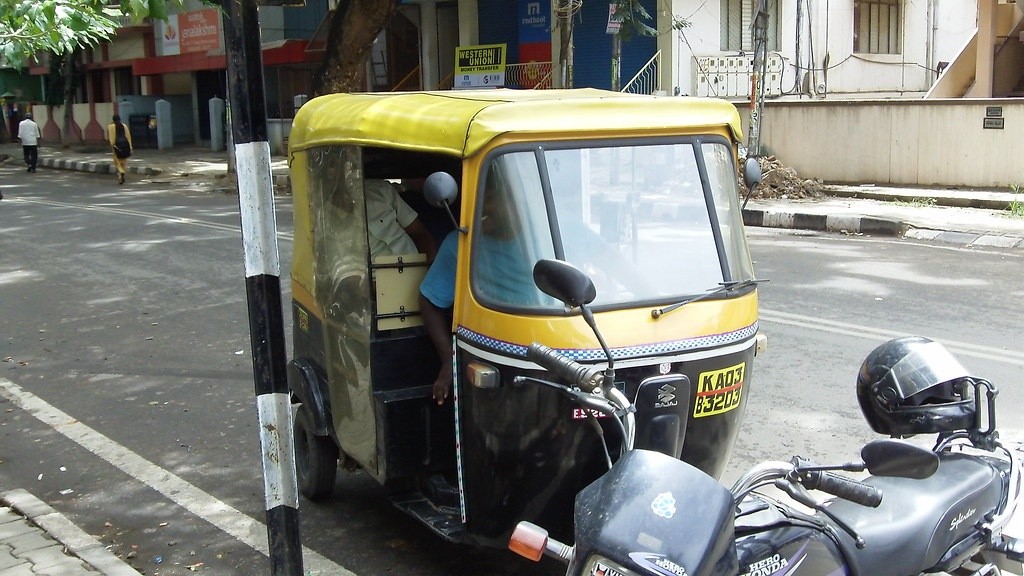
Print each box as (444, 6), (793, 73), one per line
(507, 256), (1024, 576)
(281, 83), (767, 556)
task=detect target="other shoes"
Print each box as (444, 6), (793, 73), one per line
(119, 172), (125, 184)
(27, 165), (32, 171)
(31, 170), (36, 173)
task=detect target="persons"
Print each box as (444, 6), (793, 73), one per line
(18, 112), (40, 173)
(314, 148), (437, 392)
(107, 114), (133, 184)
(419, 160), (654, 406)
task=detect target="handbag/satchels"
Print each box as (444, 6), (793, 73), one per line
(114, 135), (131, 159)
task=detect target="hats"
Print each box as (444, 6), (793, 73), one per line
(25, 113), (32, 118)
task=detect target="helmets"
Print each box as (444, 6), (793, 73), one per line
(856, 336), (966, 437)
